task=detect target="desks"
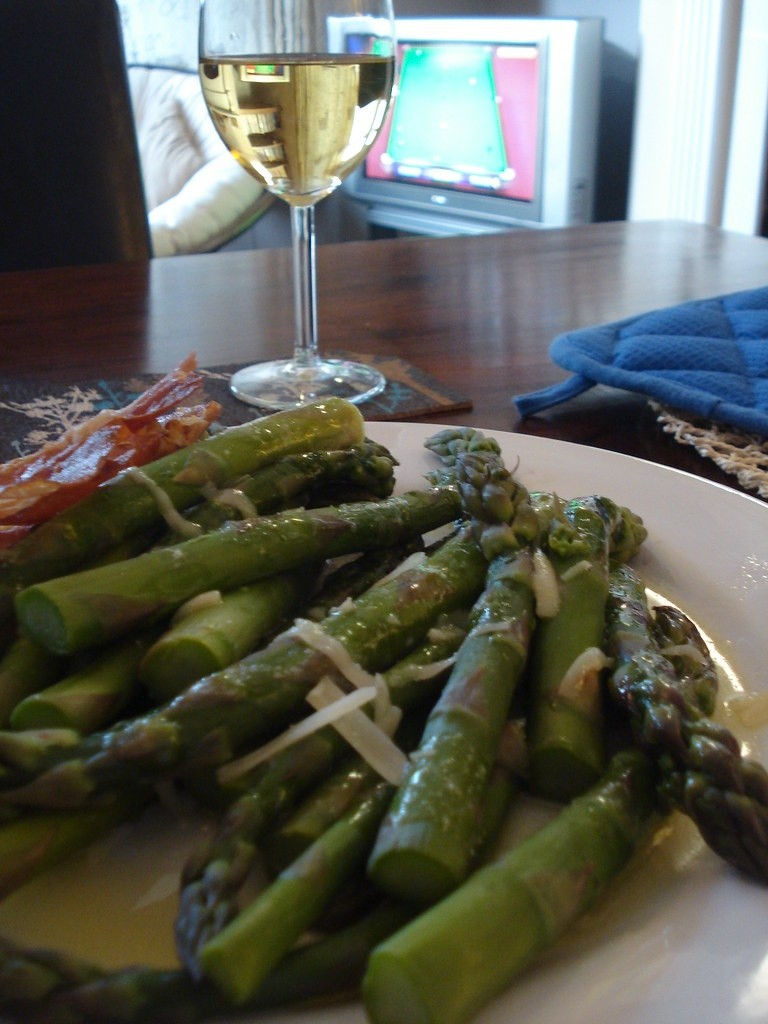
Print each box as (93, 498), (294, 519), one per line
(0, 220), (768, 503)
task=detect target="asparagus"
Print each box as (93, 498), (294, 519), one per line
(0, 396), (768, 1024)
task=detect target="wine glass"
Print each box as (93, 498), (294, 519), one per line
(198, 0), (398, 409)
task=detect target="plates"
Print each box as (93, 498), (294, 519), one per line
(0, 421), (768, 1024)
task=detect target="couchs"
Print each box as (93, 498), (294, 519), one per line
(129, 59), (277, 258)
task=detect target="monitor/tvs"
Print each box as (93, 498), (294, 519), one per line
(326, 15), (634, 238)
